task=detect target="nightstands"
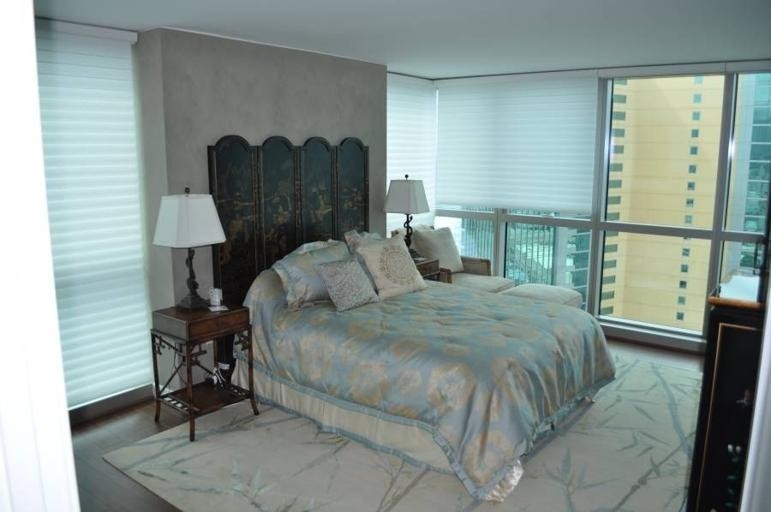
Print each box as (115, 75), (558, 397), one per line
(395, 255), (440, 284)
(149, 298), (260, 442)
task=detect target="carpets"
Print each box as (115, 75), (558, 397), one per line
(101, 331), (702, 510)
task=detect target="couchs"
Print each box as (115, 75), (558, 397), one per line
(394, 225), (584, 310)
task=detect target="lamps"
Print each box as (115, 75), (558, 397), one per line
(384, 175), (430, 262)
(153, 187), (227, 311)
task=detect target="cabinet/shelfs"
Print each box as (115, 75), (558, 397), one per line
(681, 266), (771, 506)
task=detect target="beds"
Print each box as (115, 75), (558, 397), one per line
(197, 235), (614, 488)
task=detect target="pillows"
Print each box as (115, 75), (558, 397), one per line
(271, 239), (353, 313)
(417, 227), (464, 275)
(343, 230), (394, 291)
(356, 233), (427, 300)
(315, 255), (377, 311)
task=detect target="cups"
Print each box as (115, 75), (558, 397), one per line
(209, 288), (223, 306)
